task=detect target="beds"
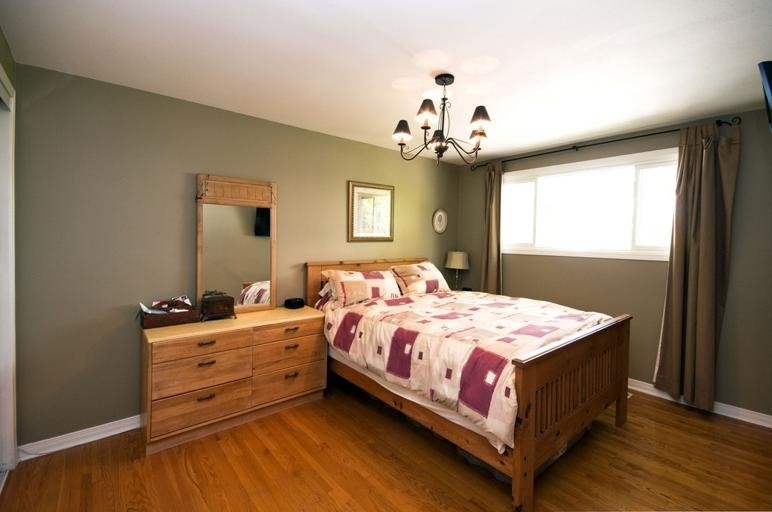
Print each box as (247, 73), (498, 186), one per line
(239, 280), (270, 305)
(303, 258), (632, 512)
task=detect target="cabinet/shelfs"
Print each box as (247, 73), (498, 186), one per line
(138, 303), (327, 458)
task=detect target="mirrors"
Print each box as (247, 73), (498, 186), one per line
(196, 173), (278, 314)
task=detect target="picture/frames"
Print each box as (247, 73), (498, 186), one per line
(432, 207), (448, 234)
(348, 179), (394, 242)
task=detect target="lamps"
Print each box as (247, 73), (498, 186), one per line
(443, 249), (470, 290)
(393, 73), (489, 166)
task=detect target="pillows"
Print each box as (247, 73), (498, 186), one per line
(329, 270), (401, 307)
(388, 261), (451, 295)
(321, 270), (341, 300)
